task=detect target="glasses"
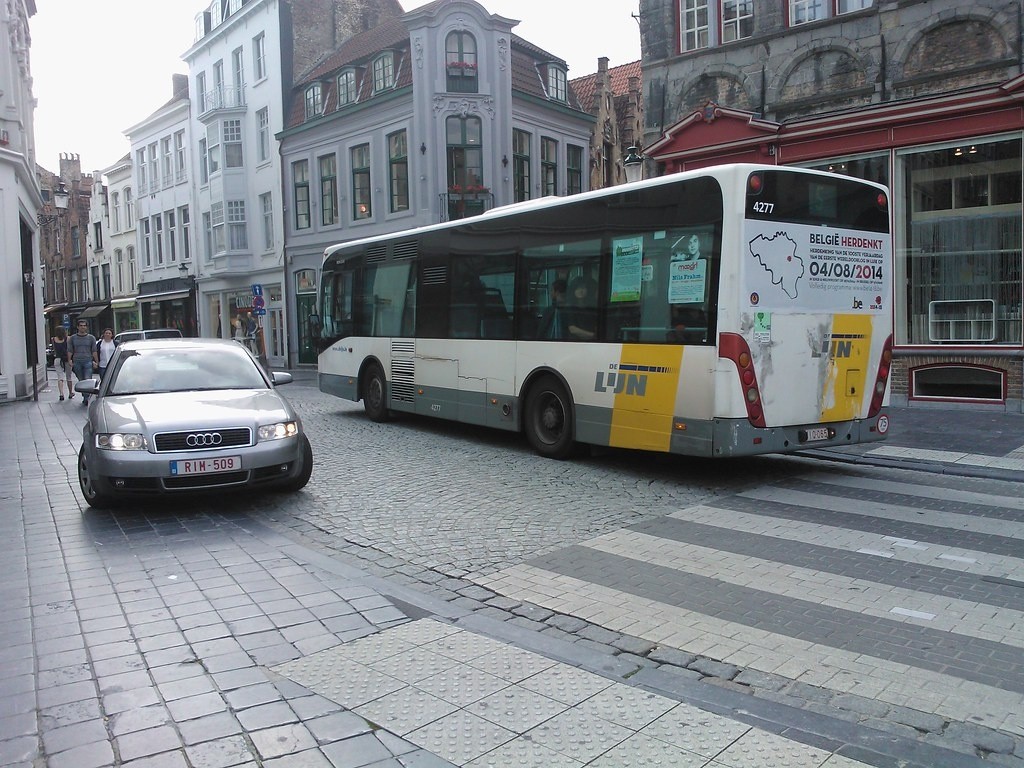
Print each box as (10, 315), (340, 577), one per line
(80, 325), (86, 327)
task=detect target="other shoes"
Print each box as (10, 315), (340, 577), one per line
(69, 393), (75, 399)
(83, 400), (88, 406)
(59, 396), (64, 400)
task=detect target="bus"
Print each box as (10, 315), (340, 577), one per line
(308, 161), (894, 461)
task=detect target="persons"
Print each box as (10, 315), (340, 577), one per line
(52, 326), (75, 401)
(675, 235), (708, 313)
(537, 265), (596, 340)
(67, 320), (98, 405)
(93, 328), (119, 380)
(236, 312), (259, 356)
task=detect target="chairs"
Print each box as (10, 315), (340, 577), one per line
(480, 287), (510, 338)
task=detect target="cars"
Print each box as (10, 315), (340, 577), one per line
(74, 327), (313, 509)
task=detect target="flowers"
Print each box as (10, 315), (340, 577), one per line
(448, 184), (463, 193)
(447, 60), (464, 68)
(464, 185), (475, 194)
(476, 185), (491, 193)
(464, 62), (478, 69)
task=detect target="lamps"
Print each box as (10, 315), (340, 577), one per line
(37, 182), (70, 226)
(178, 262), (194, 289)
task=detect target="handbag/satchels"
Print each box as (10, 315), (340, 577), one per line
(61, 356), (68, 362)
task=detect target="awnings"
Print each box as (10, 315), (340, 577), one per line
(135, 289), (192, 302)
(76, 305), (108, 318)
(43, 303), (64, 313)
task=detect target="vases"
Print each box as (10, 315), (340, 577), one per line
(449, 194), (462, 201)
(448, 68), (462, 75)
(463, 194), (476, 200)
(463, 69), (477, 76)
(476, 193), (492, 199)
